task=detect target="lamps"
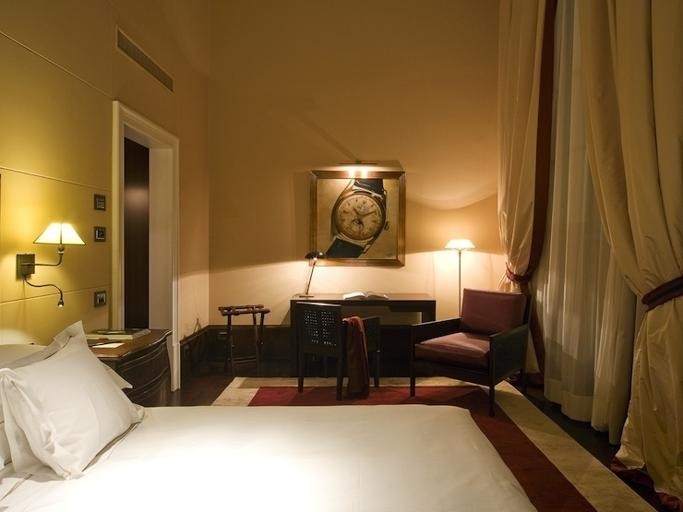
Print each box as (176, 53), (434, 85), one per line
(444, 237), (475, 319)
(17, 221), (86, 309)
(298, 251), (325, 299)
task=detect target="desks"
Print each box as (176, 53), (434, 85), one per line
(289, 293), (438, 379)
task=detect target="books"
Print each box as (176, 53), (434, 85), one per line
(343, 290), (390, 301)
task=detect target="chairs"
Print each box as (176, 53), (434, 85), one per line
(287, 299), (384, 401)
(407, 287), (533, 416)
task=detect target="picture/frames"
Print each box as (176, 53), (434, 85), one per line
(93, 289), (107, 309)
(92, 193), (107, 211)
(308, 168), (407, 270)
(93, 225), (106, 243)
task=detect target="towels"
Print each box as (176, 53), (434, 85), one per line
(342, 313), (371, 399)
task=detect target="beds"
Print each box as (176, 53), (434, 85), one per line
(1, 341), (537, 511)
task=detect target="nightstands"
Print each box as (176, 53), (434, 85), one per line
(84, 328), (174, 407)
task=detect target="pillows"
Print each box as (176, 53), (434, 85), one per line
(1, 321), (149, 481)
